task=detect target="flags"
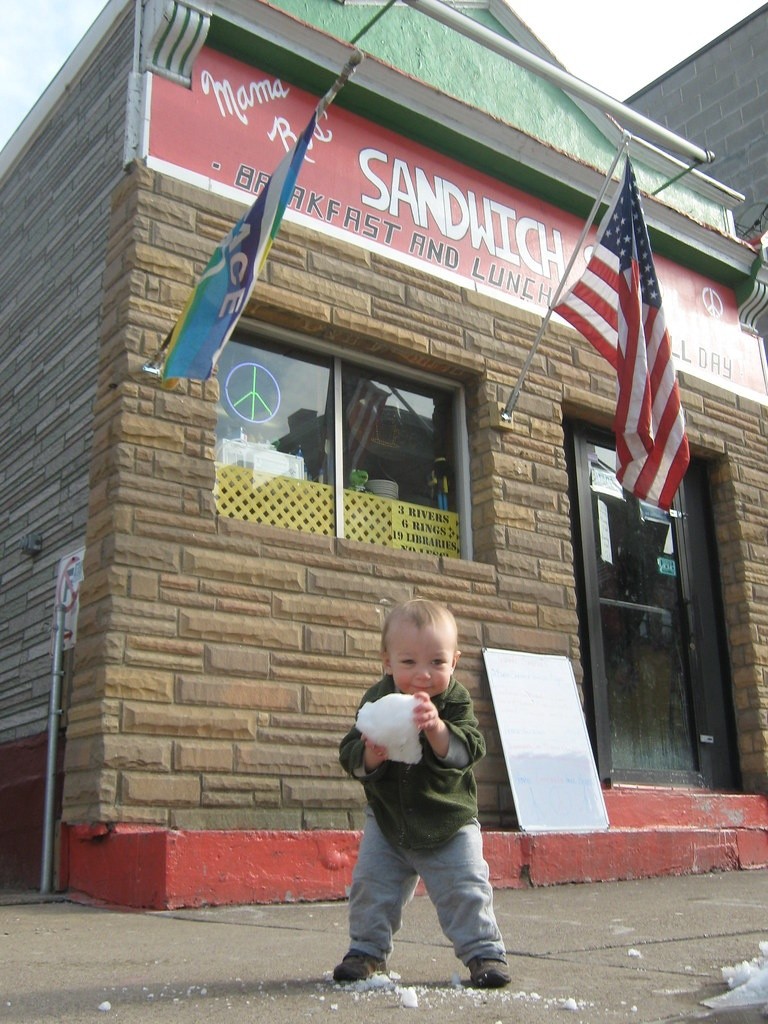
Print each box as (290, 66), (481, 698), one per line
(141, 105), (325, 383)
(553, 154), (694, 513)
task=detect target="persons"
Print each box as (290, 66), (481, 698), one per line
(328, 597), (512, 988)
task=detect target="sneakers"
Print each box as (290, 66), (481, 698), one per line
(333, 949), (389, 982)
(468, 953), (512, 989)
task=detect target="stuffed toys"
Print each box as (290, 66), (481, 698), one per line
(349, 467), (373, 497)
